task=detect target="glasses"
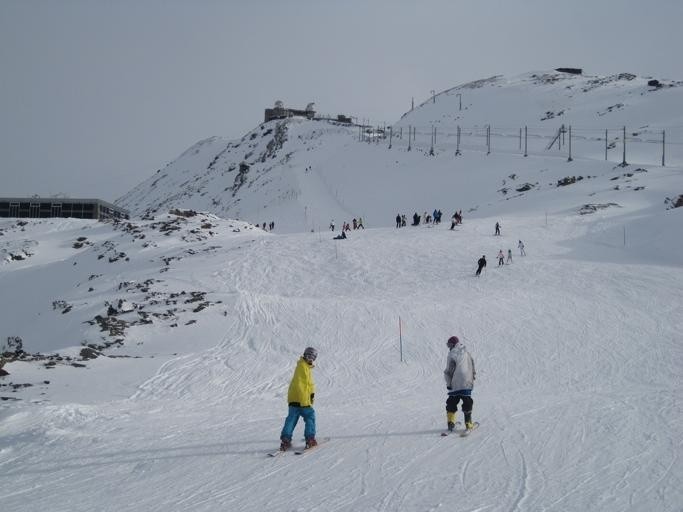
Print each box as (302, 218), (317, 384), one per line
(307, 355), (315, 362)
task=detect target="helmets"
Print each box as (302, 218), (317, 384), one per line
(447, 337), (458, 348)
(304, 348), (318, 361)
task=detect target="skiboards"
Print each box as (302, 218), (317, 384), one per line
(441, 422), (479, 437)
(267, 437), (330, 457)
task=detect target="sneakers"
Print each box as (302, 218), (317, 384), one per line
(280, 441), (290, 451)
(466, 422), (473, 429)
(307, 438), (316, 446)
(448, 422), (455, 430)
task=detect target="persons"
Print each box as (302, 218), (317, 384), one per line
(330, 208), (527, 277)
(442, 335), (476, 432)
(277, 346), (318, 452)
